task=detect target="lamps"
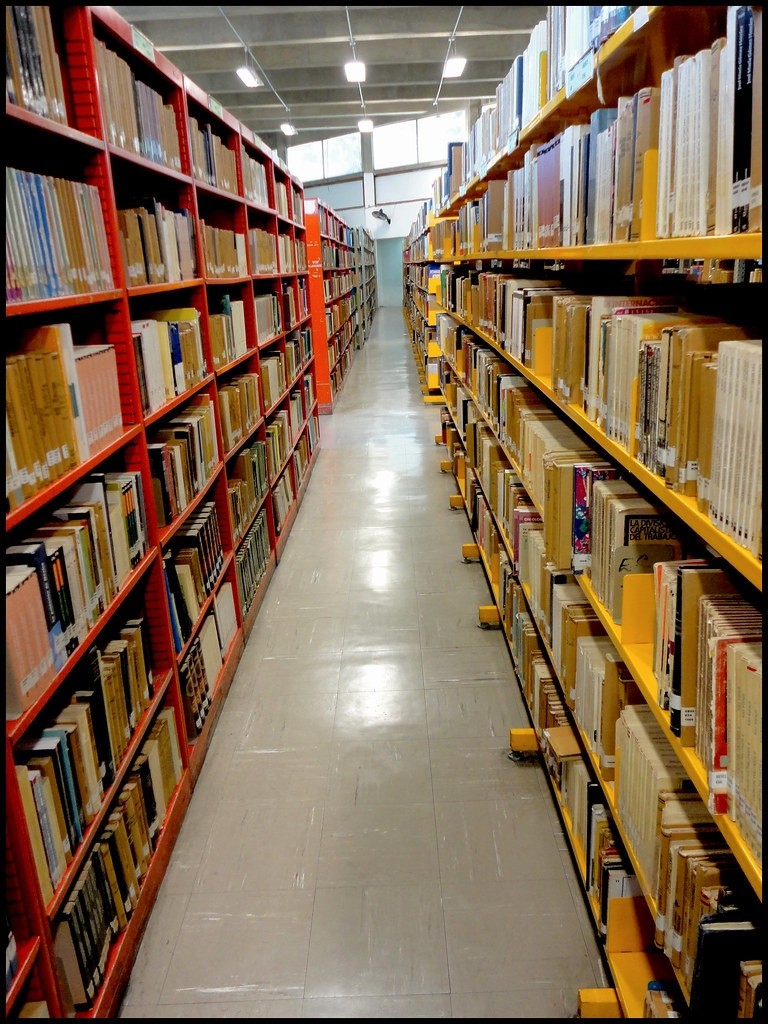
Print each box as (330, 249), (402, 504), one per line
(344, 62), (367, 83)
(358, 105), (373, 133)
(280, 108), (299, 137)
(235, 46), (265, 88)
(442, 38), (467, 78)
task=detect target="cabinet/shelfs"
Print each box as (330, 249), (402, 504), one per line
(350, 227), (365, 351)
(1, 5), (321, 1021)
(357, 224), (377, 342)
(402, 211), (446, 406)
(434, 7), (766, 1024)
(304, 197), (356, 415)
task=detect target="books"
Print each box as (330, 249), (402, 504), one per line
(6, 6), (378, 1020)
(388, 3), (764, 1023)
